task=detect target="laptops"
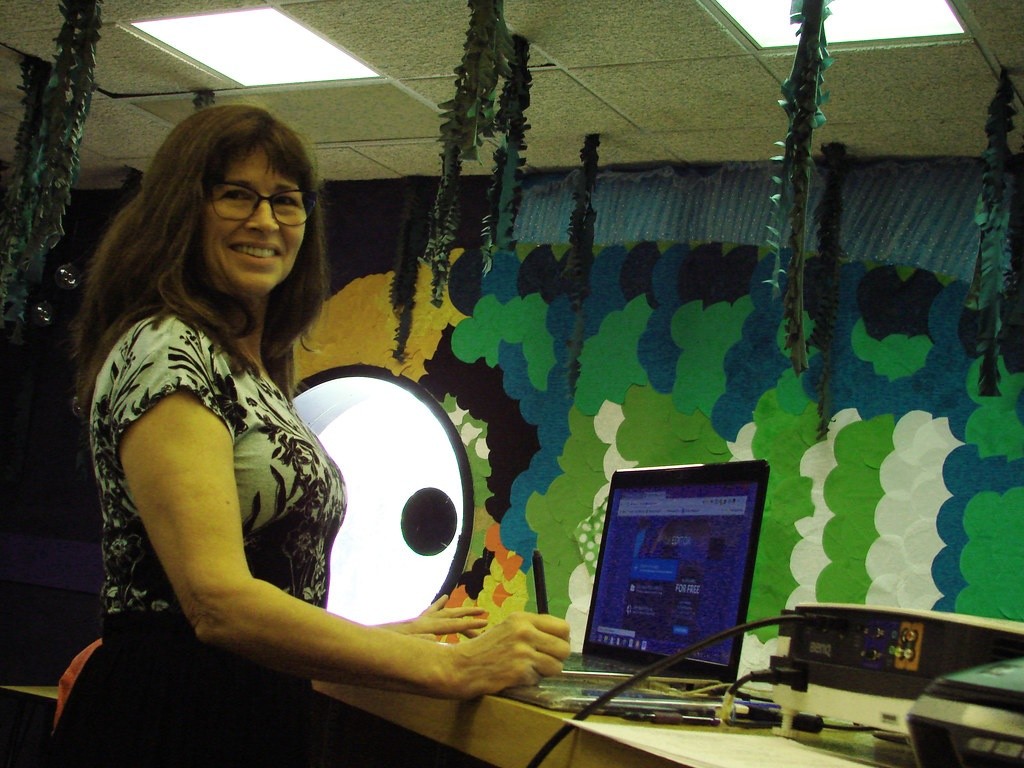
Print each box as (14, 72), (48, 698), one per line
(549, 459), (771, 691)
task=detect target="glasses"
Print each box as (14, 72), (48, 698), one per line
(205, 180), (318, 227)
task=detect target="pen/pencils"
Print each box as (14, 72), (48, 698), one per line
(532, 550), (549, 614)
(563, 692), (722, 725)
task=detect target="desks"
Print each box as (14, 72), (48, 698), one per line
(312, 654), (916, 767)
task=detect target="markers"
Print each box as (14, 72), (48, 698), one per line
(724, 702), (825, 734)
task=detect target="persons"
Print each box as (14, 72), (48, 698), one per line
(40, 105), (571, 768)
